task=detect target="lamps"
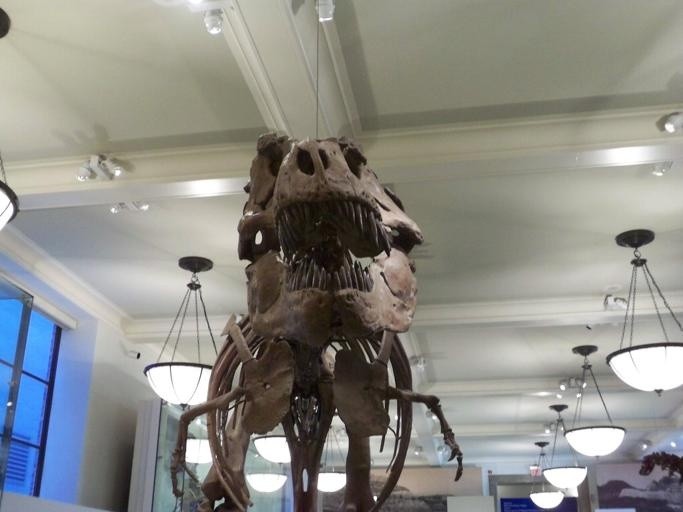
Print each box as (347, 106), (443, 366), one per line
(201, 5), (224, 36)
(665, 111), (683, 133)
(185, 424), (348, 494)
(76, 152), (124, 182)
(542, 404), (587, 490)
(143, 256), (220, 411)
(531, 442), (565, 510)
(563, 345), (626, 458)
(605, 228), (682, 396)
(315, 0), (336, 22)
